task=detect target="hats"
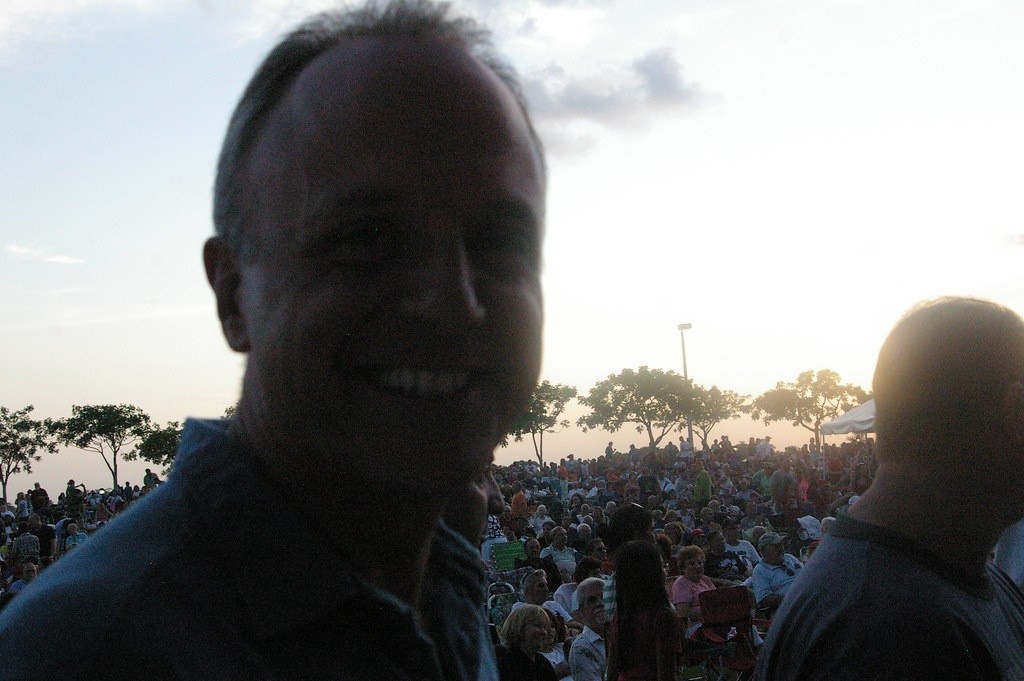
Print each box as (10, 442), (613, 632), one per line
(758, 532), (787, 547)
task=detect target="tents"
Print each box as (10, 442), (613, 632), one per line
(820, 400), (879, 480)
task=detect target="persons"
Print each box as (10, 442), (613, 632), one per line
(753, 300), (1024, 680)
(0, 437), (873, 681)
(0, 0), (546, 681)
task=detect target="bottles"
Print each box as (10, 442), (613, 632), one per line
(727, 627), (737, 641)
(555, 612), (566, 641)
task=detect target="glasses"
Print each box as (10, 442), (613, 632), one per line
(781, 563), (794, 577)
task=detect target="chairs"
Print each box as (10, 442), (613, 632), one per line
(489, 539), (529, 650)
(665, 576), (772, 681)
